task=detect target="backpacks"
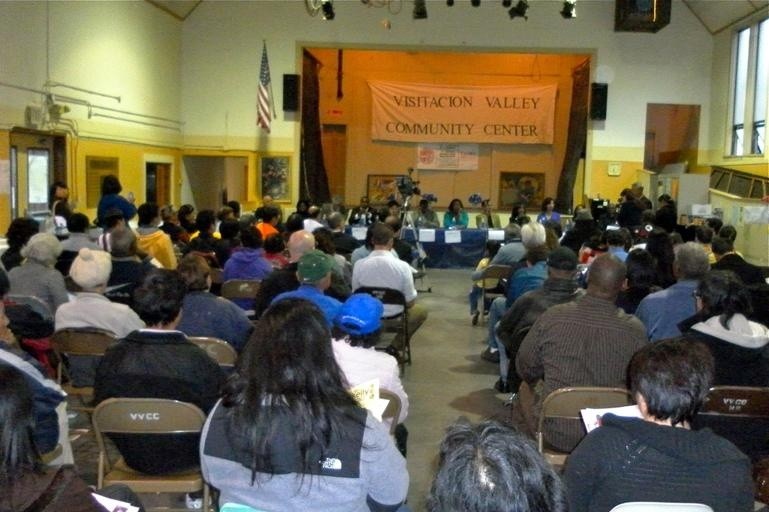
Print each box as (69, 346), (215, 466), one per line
(43, 198), (66, 234)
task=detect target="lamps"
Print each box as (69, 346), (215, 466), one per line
(302, 0), (579, 28)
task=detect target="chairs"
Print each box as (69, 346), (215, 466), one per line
(479, 235), (769, 510)
(2, 195), (413, 511)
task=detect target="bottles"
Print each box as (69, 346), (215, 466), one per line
(451, 216), (456, 230)
(481, 215), (485, 227)
(418, 217), (423, 229)
(565, 219), (570, 231)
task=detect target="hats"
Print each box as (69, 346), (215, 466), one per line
(332, 289), (386, 337)
(573, 207), (596, 223)
(295, 248), (335, 283)
(545, 243), (580, 271)
(67, 246), (114, 289)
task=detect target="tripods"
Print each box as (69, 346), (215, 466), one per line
(396, 197), (430, 292)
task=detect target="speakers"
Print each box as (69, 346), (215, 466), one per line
(283, 74), (299, 112)
(589, 81), (608, 120)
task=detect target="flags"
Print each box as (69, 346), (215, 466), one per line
(256, 44), (271, 134)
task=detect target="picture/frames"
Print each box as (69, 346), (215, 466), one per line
(257, 153), (293, 204)
(497, 170), (546, 211)
(367, 173), (412, 207)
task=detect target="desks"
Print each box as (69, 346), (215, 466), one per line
(314, 224), (562, 262)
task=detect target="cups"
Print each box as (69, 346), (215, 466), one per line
(568, 208), (571, 215)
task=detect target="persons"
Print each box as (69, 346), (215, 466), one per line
(0, 174), (427, 510)
(414, 182), (768, 511)
